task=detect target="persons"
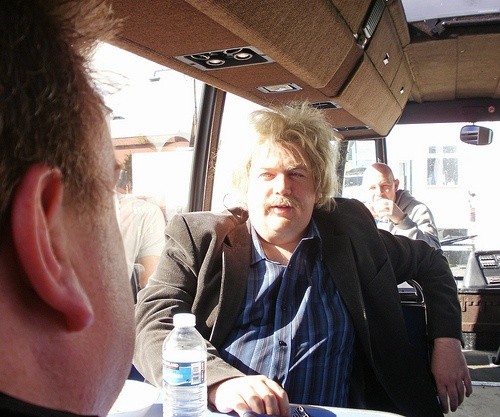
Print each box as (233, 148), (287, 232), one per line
(112, 187), (165, 292)
(133, 103), (473, 417)
(359, 163), (440, 250)
(0, 0), (135, 417)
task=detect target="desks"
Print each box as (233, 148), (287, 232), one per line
(153, 393), (404, 417)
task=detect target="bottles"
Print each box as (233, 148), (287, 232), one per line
(162, 313), (207, 417)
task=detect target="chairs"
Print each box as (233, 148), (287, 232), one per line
(396, 279), (430, 387)
